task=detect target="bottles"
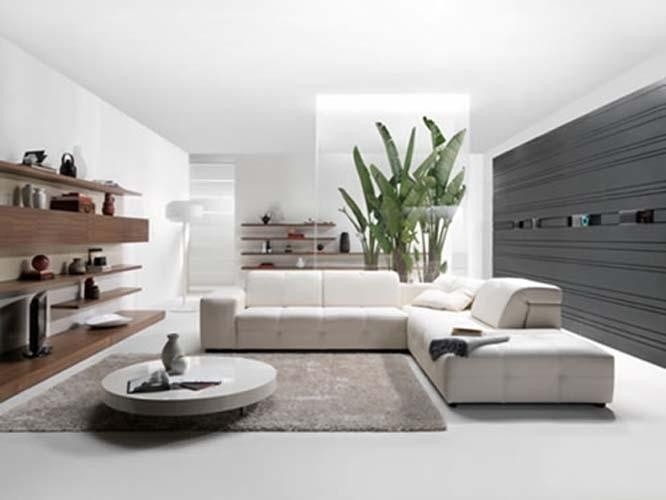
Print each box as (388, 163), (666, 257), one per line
(161, 333), (187, 374)
(23, 184), (47, 210)
(12, 185), (21, 207)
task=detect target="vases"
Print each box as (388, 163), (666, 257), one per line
(160, 333), (189, 375)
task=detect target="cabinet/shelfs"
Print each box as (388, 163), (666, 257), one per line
(1, 160), (167, 404)
(238, 221), (340, 255)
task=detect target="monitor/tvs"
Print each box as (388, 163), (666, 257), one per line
(21, 290), (49, 359)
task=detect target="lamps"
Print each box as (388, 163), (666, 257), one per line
(166, 199), (203, 312)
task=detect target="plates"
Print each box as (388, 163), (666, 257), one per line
(86, 313), (133, 330)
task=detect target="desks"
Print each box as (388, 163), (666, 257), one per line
(101, 356), (279, 419)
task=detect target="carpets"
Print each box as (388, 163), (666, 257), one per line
(1, 352), (448, 433)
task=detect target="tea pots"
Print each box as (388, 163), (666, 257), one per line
(60, 152), (77, 178)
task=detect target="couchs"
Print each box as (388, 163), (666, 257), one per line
(201, 270), (407, 353)
(401, 274), (615, 408)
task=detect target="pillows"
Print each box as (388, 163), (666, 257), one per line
(411, 272), (488, 312)
